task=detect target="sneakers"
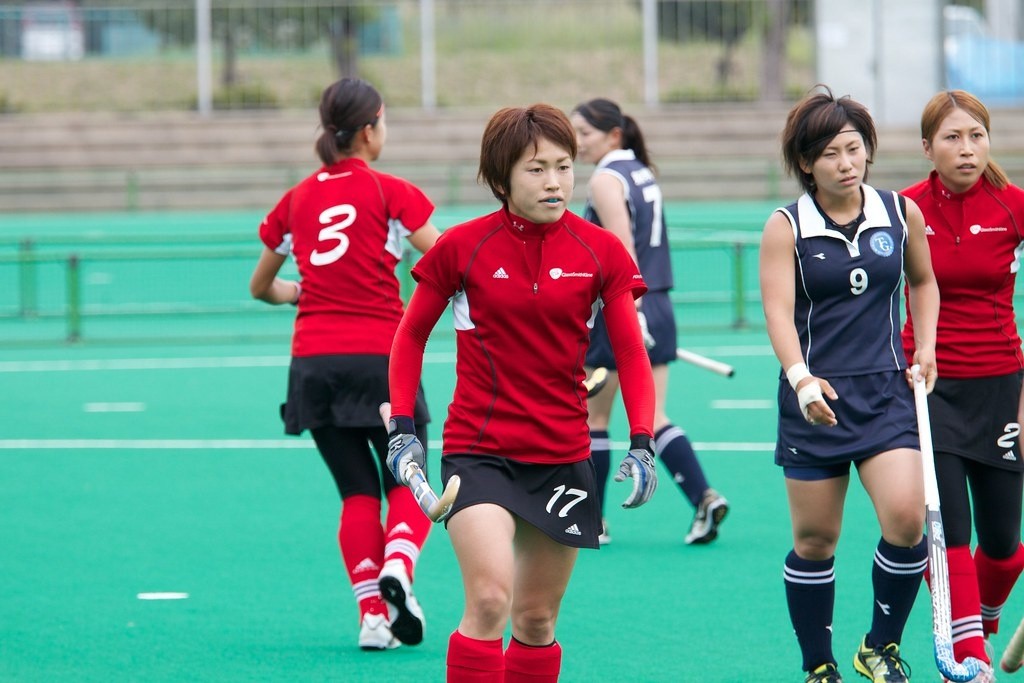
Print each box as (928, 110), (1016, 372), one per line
(597, 521), (611, 545)
(359, 616), (402, 650)
(685, 489), (729, 544)
(378, 561), (426, 647)
(805, 663), (842, 683)
(940, 641), (994, 683)
(853, 635), (911, 683)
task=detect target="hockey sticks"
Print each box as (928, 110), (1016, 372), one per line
(907, 363), (982, 683)
(377, 400), (461, 525)
(678, 348), (737, 382)
(581, 364), (610, 400)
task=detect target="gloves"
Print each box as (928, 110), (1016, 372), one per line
(614, 436), (657, 509)
(386, 415), (426, 487)
(637, 309), (655, 351)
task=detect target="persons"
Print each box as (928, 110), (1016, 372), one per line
(385, 105), (658, 683)
(569, 99), (730, 545)
(760, 93), (941, 683)
(897, 91), (1023, 683)
(249, 77), (443, 652)
